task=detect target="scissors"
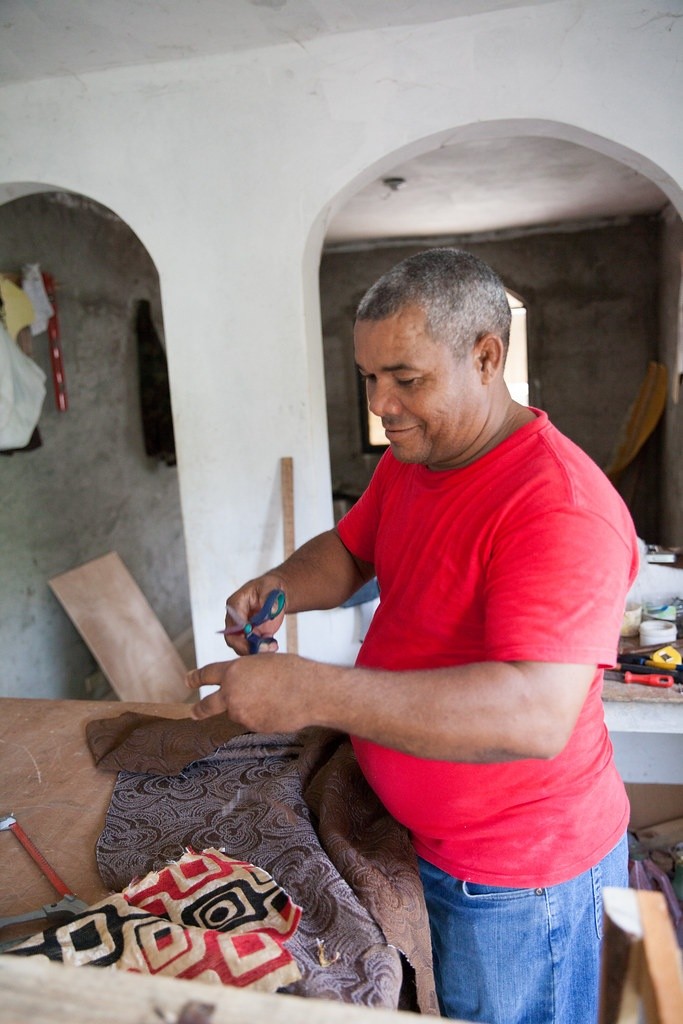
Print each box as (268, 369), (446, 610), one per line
(216, 588), (287, 655)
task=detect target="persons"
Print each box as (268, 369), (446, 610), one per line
(184, 248), (640, 1024)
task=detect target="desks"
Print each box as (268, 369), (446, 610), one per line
(599, 705), (683, 787)
(0, 695), (406, 1024)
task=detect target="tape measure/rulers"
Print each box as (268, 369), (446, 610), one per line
(653, 646), (682, 664)
